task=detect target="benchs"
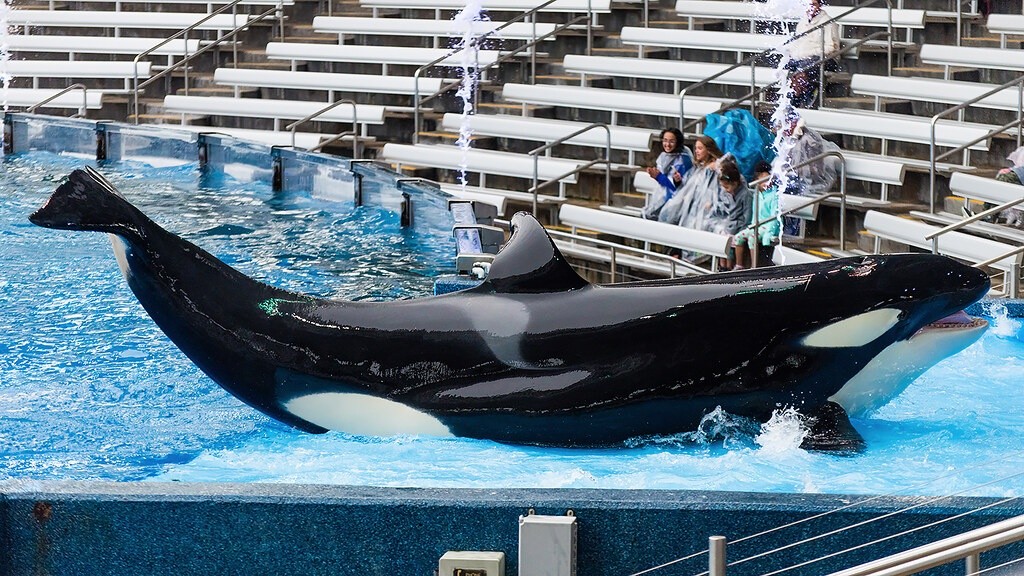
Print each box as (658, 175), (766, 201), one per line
(0, 1), (1023, 279)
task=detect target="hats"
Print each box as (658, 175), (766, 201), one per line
(720, 166), (740, 182)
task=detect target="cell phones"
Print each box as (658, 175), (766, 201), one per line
(671, 166), (678, 174)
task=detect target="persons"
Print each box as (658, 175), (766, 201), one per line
(632, 1), (842, 272)
(960, 147), (1024, 227)
